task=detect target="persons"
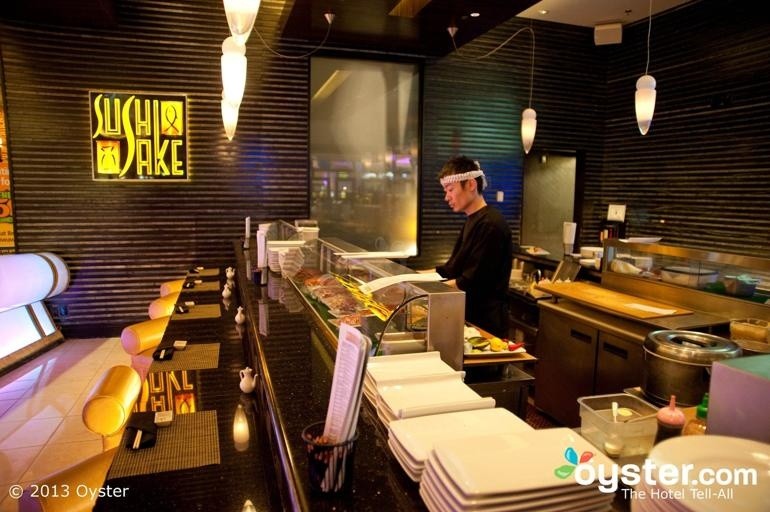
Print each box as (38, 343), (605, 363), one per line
(418, 152), (513, 338)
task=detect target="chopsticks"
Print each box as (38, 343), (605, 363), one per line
(133, 429), (142, 450)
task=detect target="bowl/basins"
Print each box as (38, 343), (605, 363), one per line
(579, 246), (603, 258)
(719, 273), (756, 297)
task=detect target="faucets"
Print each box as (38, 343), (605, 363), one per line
(529, 269), (541, 282)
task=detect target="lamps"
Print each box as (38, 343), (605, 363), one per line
(217, 0), (336, 144)
(631, 2), (663, 138)
(445, 25), (539, 156)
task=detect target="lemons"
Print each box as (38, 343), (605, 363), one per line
(490, 337), (504, 352)
(503, 342), (508, 350)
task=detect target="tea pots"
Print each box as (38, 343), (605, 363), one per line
(234, 305), (245, 325)
(224, 266), (237, 279)
(238, 365), (260, 396)
(222, 284), (231, 300)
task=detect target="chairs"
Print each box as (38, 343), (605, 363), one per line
(18, 276), (183, 510)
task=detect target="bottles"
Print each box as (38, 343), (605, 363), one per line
(654, 391), (709, 445)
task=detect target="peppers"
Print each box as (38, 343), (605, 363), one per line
(508, 341), (525, 351)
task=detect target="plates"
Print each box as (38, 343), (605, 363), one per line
(173, 340), (187, 350)
(525, 248), (550, 256)
(630, 436), (768, 511)
(154, 409), (174, 428)
(361, 347), (618, 512)
(462, 336), (527, 357)
(266, 240), (306, 274)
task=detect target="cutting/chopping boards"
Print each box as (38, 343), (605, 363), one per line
(534, 279), (694, 319)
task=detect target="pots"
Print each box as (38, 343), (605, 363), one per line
(640, 329), (744, 410)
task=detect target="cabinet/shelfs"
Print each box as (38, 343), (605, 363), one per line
(532, 298), (732, 427)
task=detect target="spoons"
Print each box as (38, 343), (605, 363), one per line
(604, 400), (623, 458)
